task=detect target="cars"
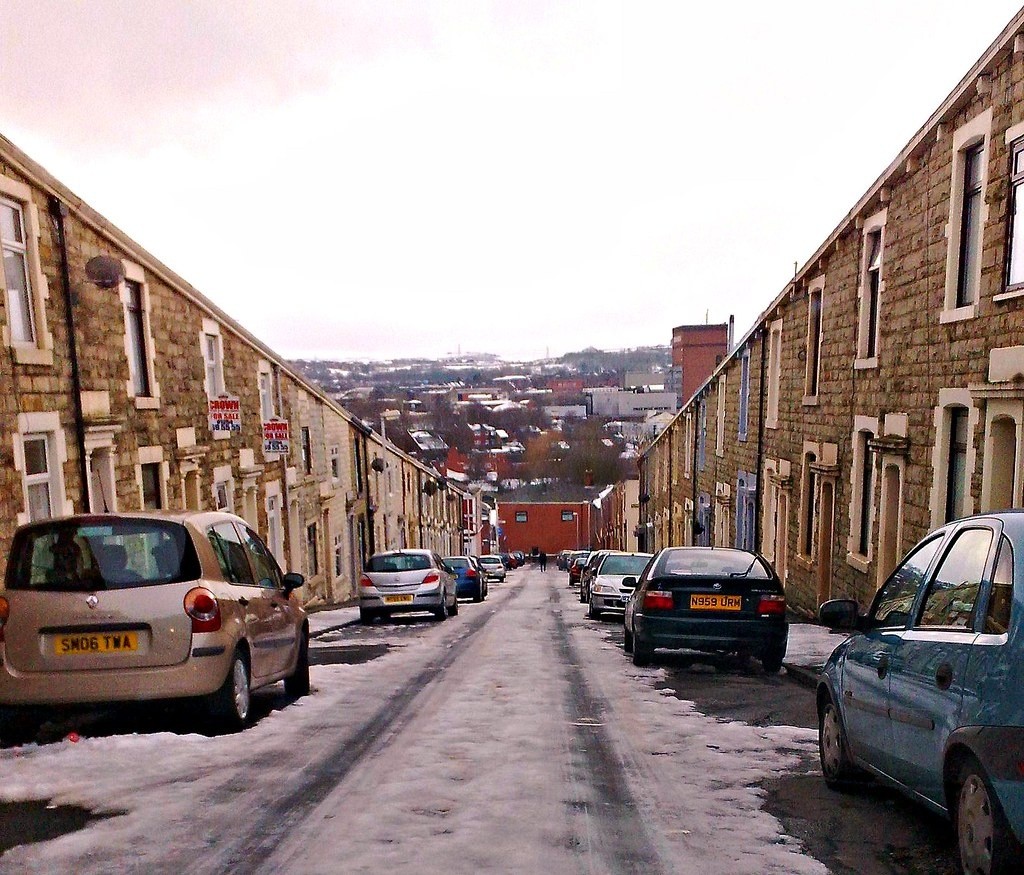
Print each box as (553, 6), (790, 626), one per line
(587, 553), (656, 620)
(356, 548), (459, 624)
(619, 545), (790, 673)
(814, 509), (1024, 875)
(0, 510), (310, 728)
(556, 549), (623, 603)
(477, 555), (506, 583)
(441, 556), (488, 603)
(495, 551), (525, 570)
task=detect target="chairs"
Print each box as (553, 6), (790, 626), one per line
(96, 545), (143, 583)
(154, 541), (179, 578)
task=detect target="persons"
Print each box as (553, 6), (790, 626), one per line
(538, 551), (547, 572)
